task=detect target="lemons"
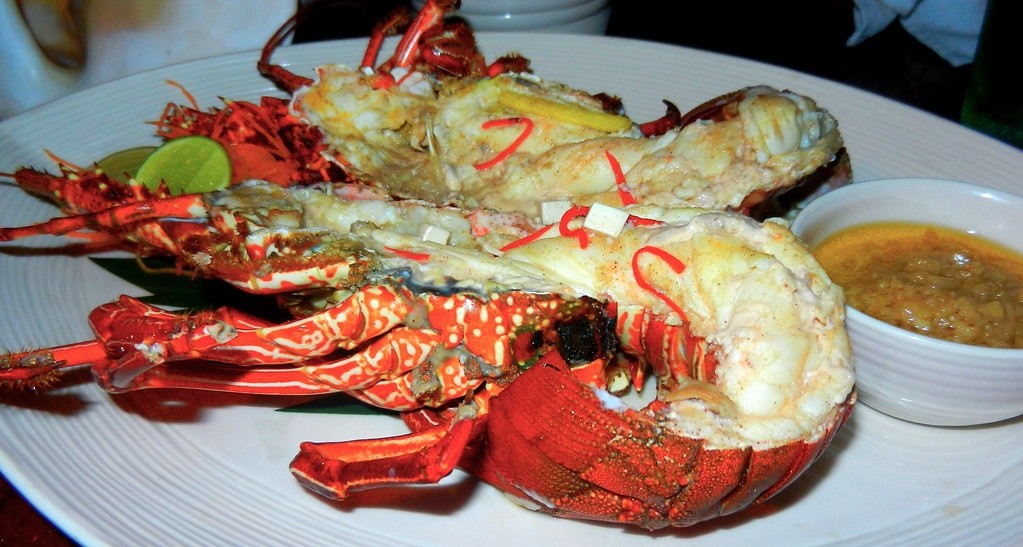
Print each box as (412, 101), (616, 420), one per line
(83, 146), (161, 184)
(130, 136), (232, 195)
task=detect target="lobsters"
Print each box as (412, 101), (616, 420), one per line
(0, 1), (858, 534)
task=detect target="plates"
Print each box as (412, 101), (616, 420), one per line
(0, 0), (299, 120)
(0, 30), (1023, 547)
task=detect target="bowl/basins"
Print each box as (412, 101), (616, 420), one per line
(414, 0), (610, 40)
(789, 176), (1023, 428)
